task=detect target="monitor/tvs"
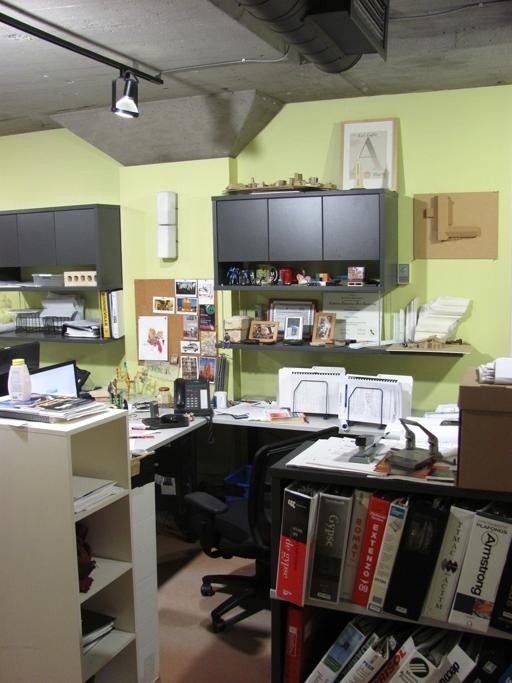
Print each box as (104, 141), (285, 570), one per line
(29, 360), (79, 398)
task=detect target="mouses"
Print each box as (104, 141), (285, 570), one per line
(161, 415), (180, 423)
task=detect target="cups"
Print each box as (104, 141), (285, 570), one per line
(279, 267), (294, 284)
(214, 391), (227, 409)
(255, 265), (278, 285)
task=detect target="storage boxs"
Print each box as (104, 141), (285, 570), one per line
(455, 365), (512, 492)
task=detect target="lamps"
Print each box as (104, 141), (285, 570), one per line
(110, 66), (140, 119)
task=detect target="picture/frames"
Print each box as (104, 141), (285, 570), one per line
(311, 312), (337, 345)
(283, 316), (304, 341)
(153, 279), (216, 385)
(338, 117), (397, 191)
(266, 299), (318, 341)
(249, 319), (279, 343)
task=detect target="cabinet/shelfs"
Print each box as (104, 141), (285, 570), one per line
(0, 203), (124, 343)
(213, 187), (400, 292)
(266, 440), (512, 683)
(0, 393), (141, 683)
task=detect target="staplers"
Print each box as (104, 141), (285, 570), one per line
(349, 435), (385, 463)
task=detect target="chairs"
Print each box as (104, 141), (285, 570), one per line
(173, 426), (340, 633)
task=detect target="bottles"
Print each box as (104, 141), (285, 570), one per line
(157, 387), (172, 415)
(8, 358), (31, 402)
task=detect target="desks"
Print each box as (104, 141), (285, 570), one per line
(211, 392), (436, 465)
(128, 395), (217, 683)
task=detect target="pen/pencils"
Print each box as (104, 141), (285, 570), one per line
(130, 436), (154, 438)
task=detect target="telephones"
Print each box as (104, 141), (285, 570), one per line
(174, 378), (211, 416)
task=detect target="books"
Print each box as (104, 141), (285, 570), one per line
(81, 606), (116, 649)
(101, 289), (125, 339)
(0, 389), (110, 423)
